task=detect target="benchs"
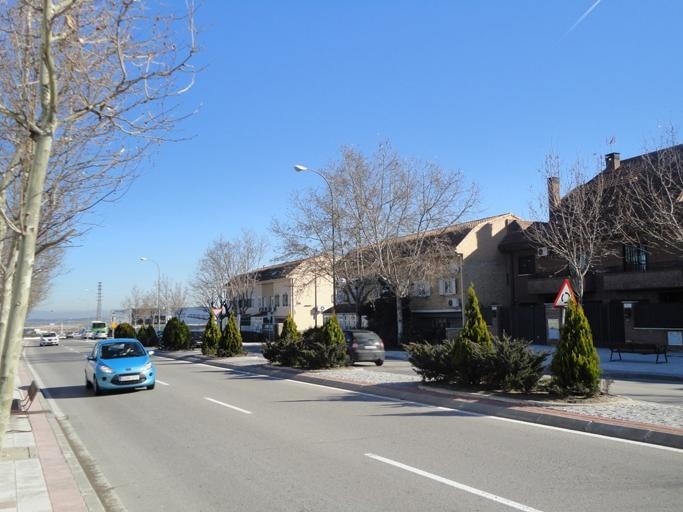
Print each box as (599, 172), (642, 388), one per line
(610, 330), (670, 364)
(11, 380), (37, 413)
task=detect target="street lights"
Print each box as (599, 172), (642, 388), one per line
(293, 166), (337, 322)
(140, 257), (161, 338)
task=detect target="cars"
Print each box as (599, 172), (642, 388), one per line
(84, 336), (155, 393)
(39, 330), (59, 347)
(94, 330), (107, 340)
(190, 336), (202, 347)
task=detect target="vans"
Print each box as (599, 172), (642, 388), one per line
(66, 330), (73, 338)
(82, 330), (94, 339)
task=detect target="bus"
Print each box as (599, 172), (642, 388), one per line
(90, 320), (109, 339)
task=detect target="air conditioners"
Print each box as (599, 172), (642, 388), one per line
(447, 298), (459, 307)
(537, 246), (551, 256)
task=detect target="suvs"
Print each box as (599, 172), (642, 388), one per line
(343, 330), (385, 367)
